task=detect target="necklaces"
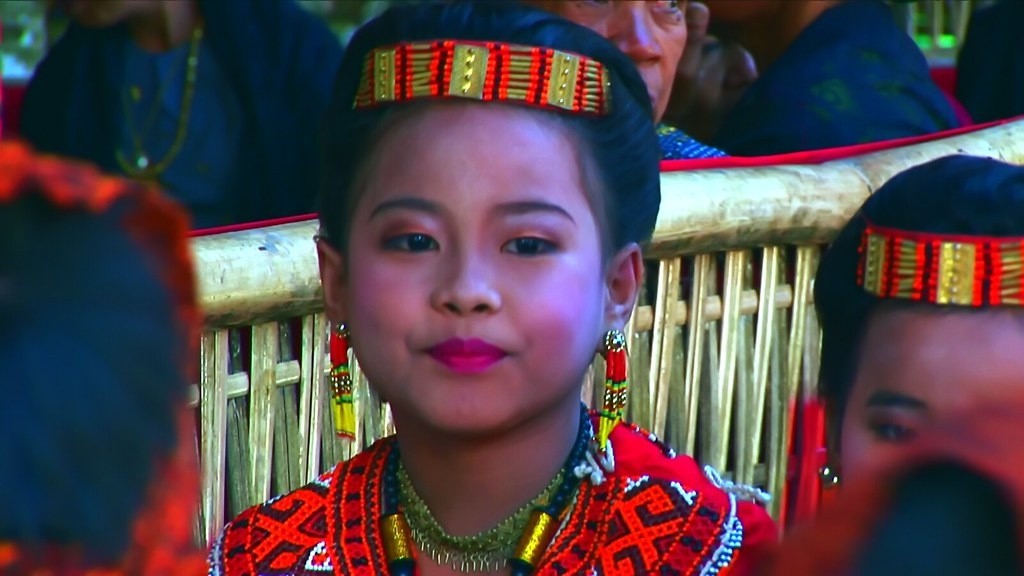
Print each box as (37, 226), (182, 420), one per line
(113, 26), (203, 179)
(377, 399), (592, 576)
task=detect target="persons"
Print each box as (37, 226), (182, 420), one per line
(202, 0), (784, 576)
(776, 152), (1024, 576)
(538, 0), (1024, 161)
(15, 0), (348, 234)
